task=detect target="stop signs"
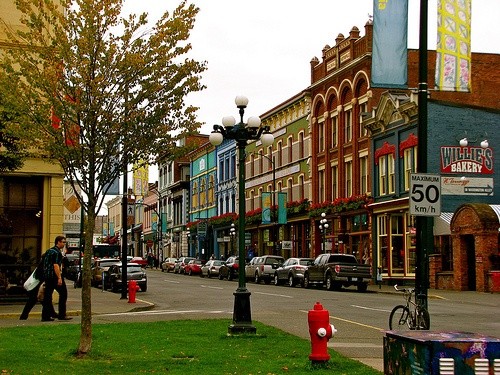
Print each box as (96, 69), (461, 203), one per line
(147, 240), (153, 247)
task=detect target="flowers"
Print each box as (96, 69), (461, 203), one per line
(186, 198), (312, 232)
(305, 194), (374, 216)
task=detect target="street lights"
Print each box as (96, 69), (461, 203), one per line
(185, 96), (329, 333)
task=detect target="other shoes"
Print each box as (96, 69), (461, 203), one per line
(42, 317), (55, 322)
(58, 317), (73, 321)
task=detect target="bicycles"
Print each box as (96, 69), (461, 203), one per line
(389, 284), (430, 330)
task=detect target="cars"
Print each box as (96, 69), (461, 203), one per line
(162, 258), (180, 272)
(201, 260), (226, 278)
(127, 256), (133, 260)
(130, 256), (147, 266)
(104, 263), (147, 293)
(66, 251), (84, 280)
(183, 259), (205, 275)
(218, 256), (252, 280)
(274, 258), (315, 287)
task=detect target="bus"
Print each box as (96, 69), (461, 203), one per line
(92, 243), (111, 258)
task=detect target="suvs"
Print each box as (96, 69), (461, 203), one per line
(91, 259), (122, 288)
(173, 257), (196, 274)
(245, 255), (286, 284)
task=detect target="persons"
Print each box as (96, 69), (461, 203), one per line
(20, 255), (57, 320)
(43, 236), (67, 322)
(146, 252), (154, 268)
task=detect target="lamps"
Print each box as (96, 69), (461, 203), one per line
(459, 138), (468, 147)
(480, 140), (489, 148)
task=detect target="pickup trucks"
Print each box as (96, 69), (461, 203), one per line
(303, 254), (373, 291)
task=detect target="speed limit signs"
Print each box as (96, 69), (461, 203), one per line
(409, 172), (441, 216)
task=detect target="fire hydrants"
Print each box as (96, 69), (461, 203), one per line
(127, 280), (140, 303)
(308, 302), (337, 360)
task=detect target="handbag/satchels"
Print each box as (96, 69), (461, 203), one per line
(24, 275), (39, 291)
